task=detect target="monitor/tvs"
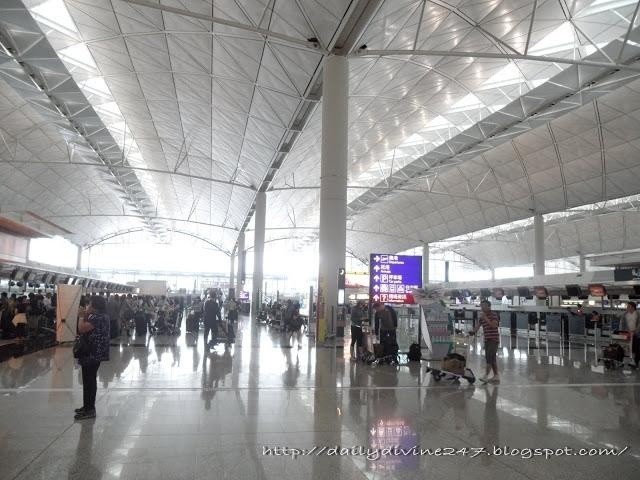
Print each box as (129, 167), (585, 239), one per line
(450, 283), (607, 298)
(10, 268), (133, 292)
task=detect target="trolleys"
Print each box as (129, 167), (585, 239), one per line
(427, 327), (476, 383)
(374, 326), (398, 366)
(597, 329), (633, 369)
(210, 320), (235, 347)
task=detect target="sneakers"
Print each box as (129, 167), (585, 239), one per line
(74, 406), (96, 420)
(478, 374), (489, 383)
(488, 374), (500, 381)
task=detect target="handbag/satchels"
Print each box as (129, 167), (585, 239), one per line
(373, 339), (385, 357)
(441, 353), (466, 374)
(73, 335), (90, 358)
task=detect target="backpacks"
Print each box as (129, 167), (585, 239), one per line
(407, 342), (421, 363)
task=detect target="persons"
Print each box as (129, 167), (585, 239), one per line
(469, 300), (500, 383)
(262, 298), (300, 332)
(74, 295), (110, 420)
(8, 340), (56, 388)
(480, 381), (499, 466)
(349, 360), (365, 424)
(618, 302), (640, 370)
(350, 300), (368, 361)
(376, 363), (399, 423)
(373, 303), (397, 363)
(68, 417), (102, 480)
(268, 326), (301, 388)
(0, 289), (57, 340)
(80, 292), (237, 349)
(98, 331), (232, 410)
